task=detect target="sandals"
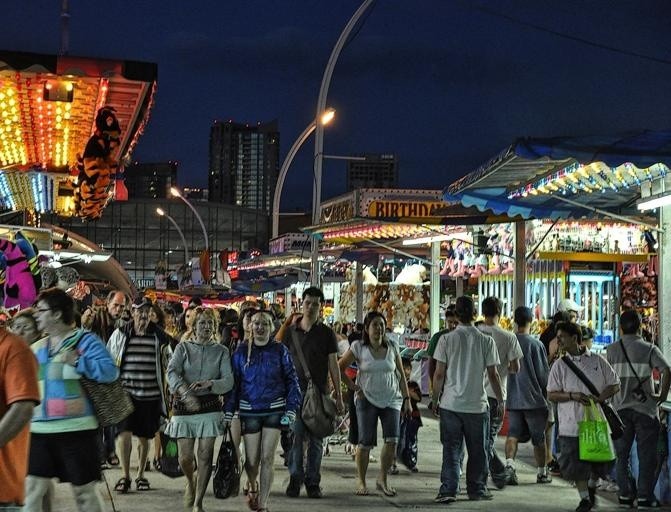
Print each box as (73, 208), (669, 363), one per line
(145, 461), (151, 471)
(114, 477), (132, 493)
(135, 478), (149, 491)
(152, 458), (161, 471)
(106, 454), (120, 465)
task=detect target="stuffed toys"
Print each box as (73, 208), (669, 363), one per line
(66, 108), (124, 223)
(436, 229), (518, 279)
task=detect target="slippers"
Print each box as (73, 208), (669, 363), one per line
(247, 480), (260, 511)
(376, 480), (397, 497)
(352, 488), (368, 496)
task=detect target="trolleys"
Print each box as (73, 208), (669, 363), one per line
(324, 386), (364, 460)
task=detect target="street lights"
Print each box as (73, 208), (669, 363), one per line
(261, 104), (338, 250)
(166, 186), (210, 264)
(153, 207), (190, 271)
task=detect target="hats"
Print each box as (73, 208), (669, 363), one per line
(132, 296), (154, 310)
(455, 296), (476, 316)
(557, 299), (585, 312)
(515, 306), (533, 318)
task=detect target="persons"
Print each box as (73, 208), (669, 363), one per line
(2, 285), (670, 511)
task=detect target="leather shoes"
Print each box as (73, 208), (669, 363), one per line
(286, 474), (303, 496)
(305, 483), (322, 498)
(388, 464), (399, 476)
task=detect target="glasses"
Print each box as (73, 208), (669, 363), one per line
(34, 308), (53, 314)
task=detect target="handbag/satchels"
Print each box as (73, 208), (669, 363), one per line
(300, 381), (337, 438)
(398, 414), (421, 469)
(213, 422), (241, 498)
(168, 382), (223, 416)
(73, 332), (137, 429)
(158, 423), (197, 478)
(599, 399), (626, 441)
(578, 399), (617, 463)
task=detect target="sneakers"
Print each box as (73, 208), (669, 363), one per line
(183, 476), (196, 508)
(434, 461), (663, 511)
